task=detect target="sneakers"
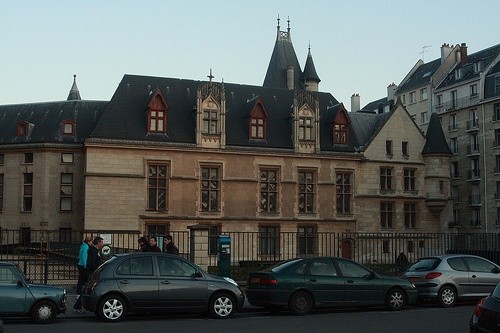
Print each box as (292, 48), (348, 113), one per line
(72, 309), (83, 314)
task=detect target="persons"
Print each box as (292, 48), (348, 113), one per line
(76, 236), (93, 301)
(162, 234), (179, 255)
(137, 236), (154, 252)
(72, 236), (105, 314)
(149, 235), (162, 252)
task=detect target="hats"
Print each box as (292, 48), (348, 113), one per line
(138, 237), (147, 243)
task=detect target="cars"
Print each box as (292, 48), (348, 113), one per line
(245, 256), (420, 316)
(404, 254), (500, 307)
(83, 252), (244, 322)
(469, 282), (500, 333)
(0, 261), (67, 324)
